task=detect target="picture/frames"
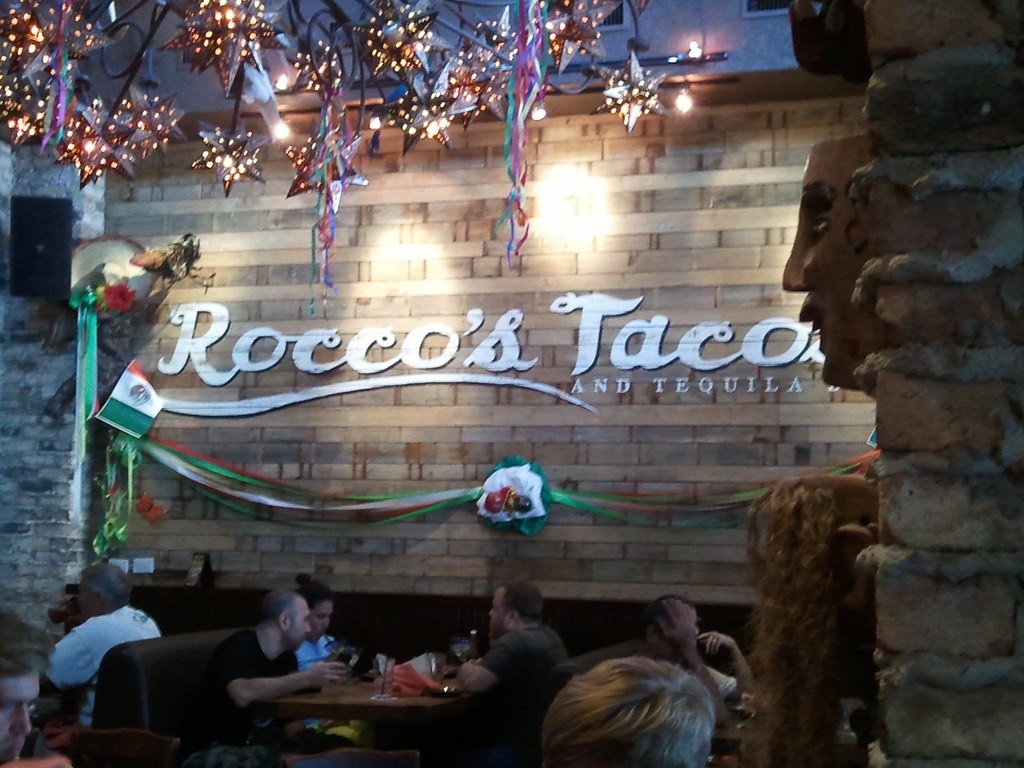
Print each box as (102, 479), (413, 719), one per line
(184, 552), (216, 587)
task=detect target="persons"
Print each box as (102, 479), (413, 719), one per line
(0, 610), (85, 768)
(40, 559), (160, 730)
(443, 578), (568, 768)
(180, 572), (377, 768)
(538, 476), (878, 768)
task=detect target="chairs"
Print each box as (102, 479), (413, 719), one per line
(70, 729), (180, 768)
(279, 747), (421, 768)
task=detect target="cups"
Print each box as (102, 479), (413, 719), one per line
(372, 658), (395, 701)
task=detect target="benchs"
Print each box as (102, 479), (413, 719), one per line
(548, 637), (644, 701)
(91, 625), (259, 768)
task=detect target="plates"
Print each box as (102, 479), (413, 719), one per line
(428, 686), (465, 697)
(358, 672), (378, 681)
(443, 670), (459, 678)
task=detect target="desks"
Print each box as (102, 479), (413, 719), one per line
(709, 695), (868, 768)
(254, 682), (474, 746)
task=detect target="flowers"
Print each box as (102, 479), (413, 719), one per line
(94, 283), (137, 316)
